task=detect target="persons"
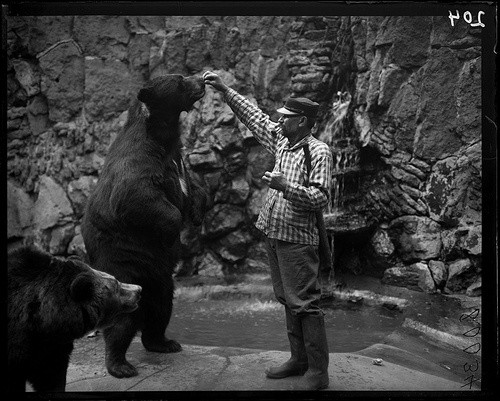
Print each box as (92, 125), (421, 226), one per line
(200, 71), (334, 392)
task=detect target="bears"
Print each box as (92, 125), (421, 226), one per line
(7, 242), (143, 391)
(79, 72), (207, 379)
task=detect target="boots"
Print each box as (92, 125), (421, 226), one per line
(265, 311), (308, 379)
(293, 314), (329, 390)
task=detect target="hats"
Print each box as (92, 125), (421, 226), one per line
(277, 98), (320, 116)
